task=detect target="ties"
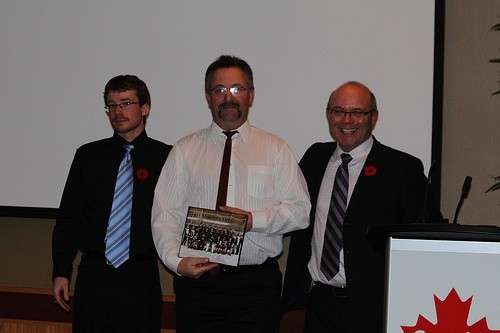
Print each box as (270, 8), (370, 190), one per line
(215, 131), (239, 212)
(105, 144), (134, 269)
(320, 153), (353, 282)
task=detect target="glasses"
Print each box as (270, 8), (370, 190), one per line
(206, 85), (250, 97)
(104, 101), (143, 116)
(327, 106), (376, 122)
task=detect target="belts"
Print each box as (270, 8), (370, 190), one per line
(105, 257), (135, 265)
(219, 258), (276, 274)
(313, 284), (348, 297)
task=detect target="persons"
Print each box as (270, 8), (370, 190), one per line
(51, 74), (175, 333)
(150, 55), (312, 333)
(281, 81), (445, 333)
(181, 224), (240, 256)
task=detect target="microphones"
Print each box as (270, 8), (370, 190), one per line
(453, 176), (473, 224)
